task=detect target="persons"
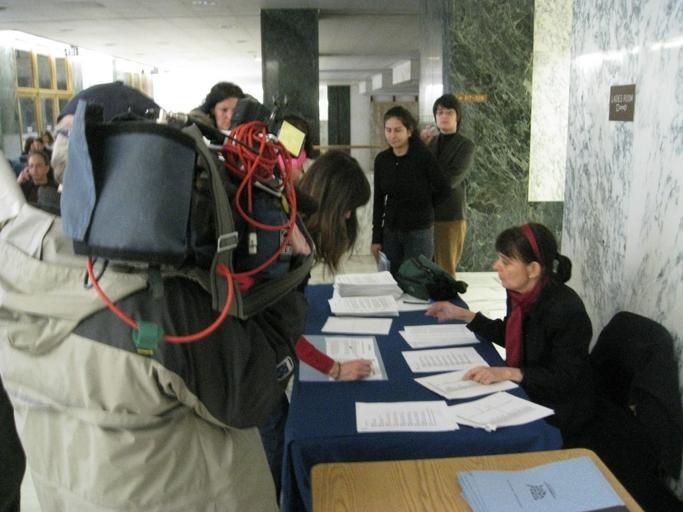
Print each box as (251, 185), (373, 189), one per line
(415, 93), (473, 277)
(422, 222), (595, 441)
(236, 147), (375, 502)
(13, 81), (308, 212)
(0, 80), (290, 511)
(369, 104), (449, 277)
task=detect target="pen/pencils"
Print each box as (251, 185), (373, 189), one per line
(348, 346), (375, 374)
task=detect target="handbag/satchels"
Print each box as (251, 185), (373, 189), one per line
(396, 253), (468, 303)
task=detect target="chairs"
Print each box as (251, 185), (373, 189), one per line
(569, 310), (682, 502)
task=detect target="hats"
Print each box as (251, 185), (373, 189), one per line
(57, 80), (162, 130)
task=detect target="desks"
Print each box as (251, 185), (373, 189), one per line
(308, 445), (646, 512)
(270, 281), (563, 512)
